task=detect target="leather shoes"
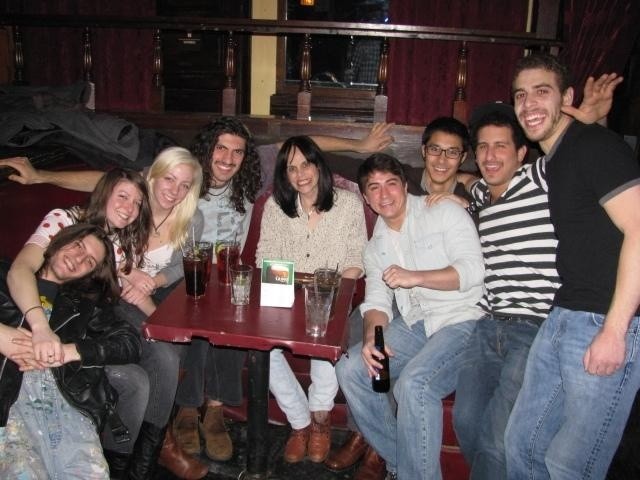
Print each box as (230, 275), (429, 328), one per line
(308, 411), (331, 463)
(324, 430), (367, 472)
(172, 406), (202, 457)
(353, 446), (387, 480)
(200, 405), (233, 462)
(285, 423), (308, 463)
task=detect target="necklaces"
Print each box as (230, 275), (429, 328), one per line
(306, 207), (318, 220)
(206, 179), (231, 202)
(149, 206), (175, 237)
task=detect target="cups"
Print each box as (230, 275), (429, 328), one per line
(303, 279), (335, 339)
(313, 267), (343, 323)
(215, 239), (242, 287)
(227, 263), (254, 307)
(188, 239), (213, 291)
(182, 252), (209, 298)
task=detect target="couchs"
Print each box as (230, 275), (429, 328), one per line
(178, 170), (471, 480)
(0, 165), (114, 267)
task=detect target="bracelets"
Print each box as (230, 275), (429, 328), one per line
(21, 305), (43, 315)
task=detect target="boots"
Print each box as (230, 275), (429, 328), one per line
(103, 448), (132, 480)
(159, 424), (208, 479)
(131, 420), (168, 479)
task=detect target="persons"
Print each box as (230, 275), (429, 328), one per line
(138, 146), (209, 480)
(502, 54), (640, 480)
(0, 224), (143, 480)
(337, 152), (484, 480)
(0, 117), (395, 462)
(450, 74), (622, 479)
(309, 116), (473, 479)
(257, 135), (367, 461)
(7, 167), (180, 480)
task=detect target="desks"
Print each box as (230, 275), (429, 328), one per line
(141, 266), (359, 480)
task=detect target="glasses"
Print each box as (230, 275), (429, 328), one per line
(424, 143), (464, 158)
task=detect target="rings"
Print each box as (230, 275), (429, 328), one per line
(141, 283), (148, 289)
(46, 353), (55, 358)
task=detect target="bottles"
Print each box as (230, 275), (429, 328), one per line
(371, 325), (390, 393)
